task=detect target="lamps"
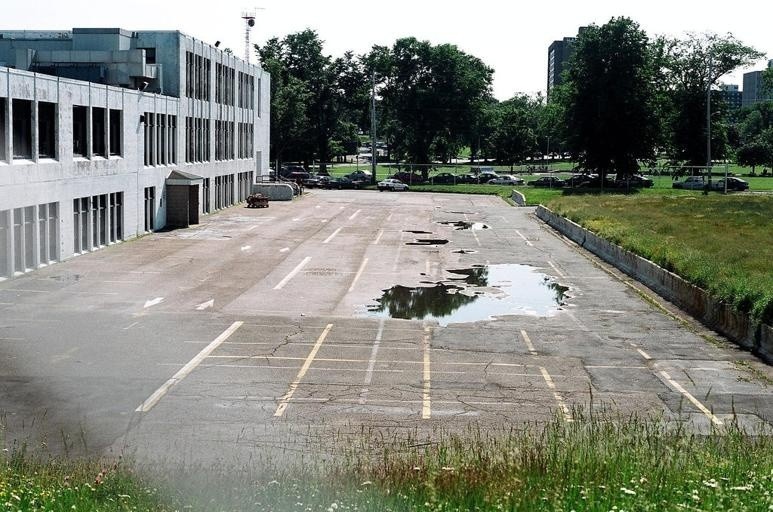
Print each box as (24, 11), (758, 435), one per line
(139, 81), (149, 91)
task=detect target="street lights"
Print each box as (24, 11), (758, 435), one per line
(476, 134), (485, 184)
(545, 135), (552, 173)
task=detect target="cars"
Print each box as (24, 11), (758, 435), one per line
(428, 171), (526, 186)
(527, 174), (655, 188)
(269, 162), (309, 195)
(377, 172), (424, 192)
(344, 170), (373, 183)
(303, 176), (359, 190)
(672, 176), (749, 191)
